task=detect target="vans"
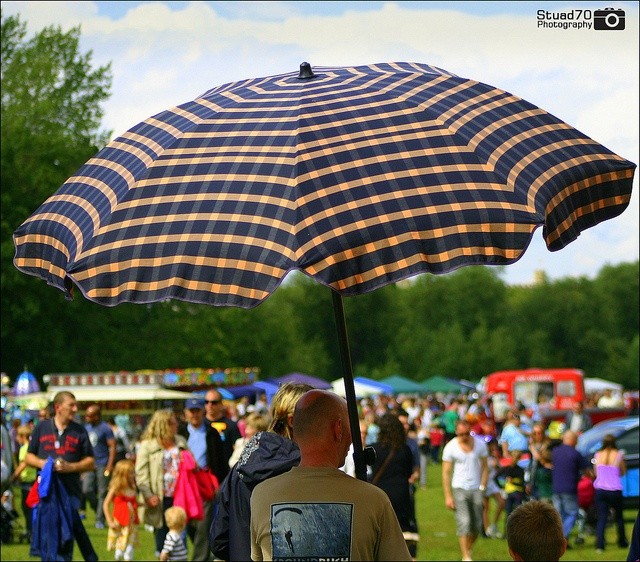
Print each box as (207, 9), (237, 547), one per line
(482, 368), (586, 420)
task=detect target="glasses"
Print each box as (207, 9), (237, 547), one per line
(455, 432), (471, 437)
(203, 398), (221, 405)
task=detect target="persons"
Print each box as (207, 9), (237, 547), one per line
(103, 460), (146, 562)
(507, 502), (567, 562)
(480, 435), (504, 543)
(395, 408), (421, 558)
(552, 431), (595, 546)
(529, 425), (553, 505)
(158, 506), (189, 560)
(470, 398), (496, 435)
(249, 389), (413, 562)
(339, 416), (373, 481)
(596, 435), (629, 552)
(598, 388), (623, 408)
(208, 379), (316, 562)
(36, 408), (50, 421)
(105, 416), (129, 453)
(429, 400), (460, 444)
(442, 421), (491, 562)
(204, 389), (242, 458)
(565, 401), (593, 431)
(0, 418), (13, 544)
(9, 424), (37, 544)
(430, 418), (446, 462)
(176, 398), (231, 562)
(134, 407), (200, 562)
(82, 403), (119, 526)
(498, 413), (531, 457)
(27, 391), (99, 562)
(228, 413), (269, 467)
(365, 412), (419, 557)
(494, 451), (529, 514)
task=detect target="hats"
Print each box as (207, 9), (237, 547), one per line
(185, 397), (204, 411)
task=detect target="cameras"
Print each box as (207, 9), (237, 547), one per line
(593, 8), (627, 31)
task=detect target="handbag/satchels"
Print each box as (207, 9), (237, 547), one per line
(173, 463), (221, 502)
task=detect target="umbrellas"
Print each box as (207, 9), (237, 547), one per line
(11, 60), (637, 480)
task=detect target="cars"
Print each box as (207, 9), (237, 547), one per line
(575, 415), (640, 528)
